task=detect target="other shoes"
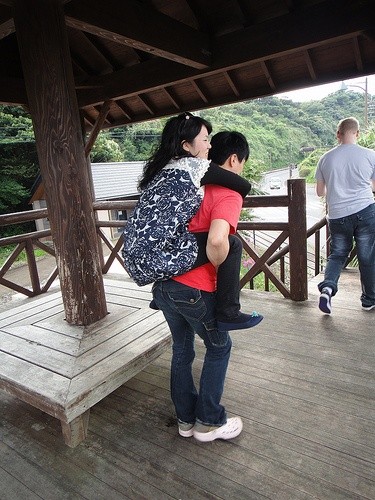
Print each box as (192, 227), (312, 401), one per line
(217, 312), (263, 332)
(361, 302), (375, 311)
(149, 300), (160, 310)
(319, 289), (333, 314)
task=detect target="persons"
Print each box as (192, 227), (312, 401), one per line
(315, 117), (375, 313)
(121, 112), (263, 332)
(151, 130), (250, 443)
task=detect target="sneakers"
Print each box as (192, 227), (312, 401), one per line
(178, 416), (243, 441)
